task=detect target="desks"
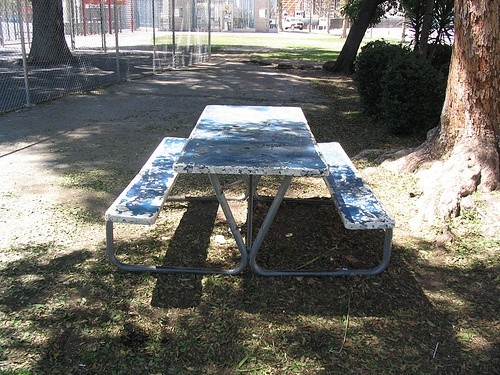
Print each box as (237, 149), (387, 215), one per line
(172, 104), (329, 275)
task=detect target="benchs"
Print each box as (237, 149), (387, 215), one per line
(314, 142), (395, 275)
(105, 136), (188, 272)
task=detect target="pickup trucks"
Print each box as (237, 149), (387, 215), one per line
(281, 17), (304, 31)
